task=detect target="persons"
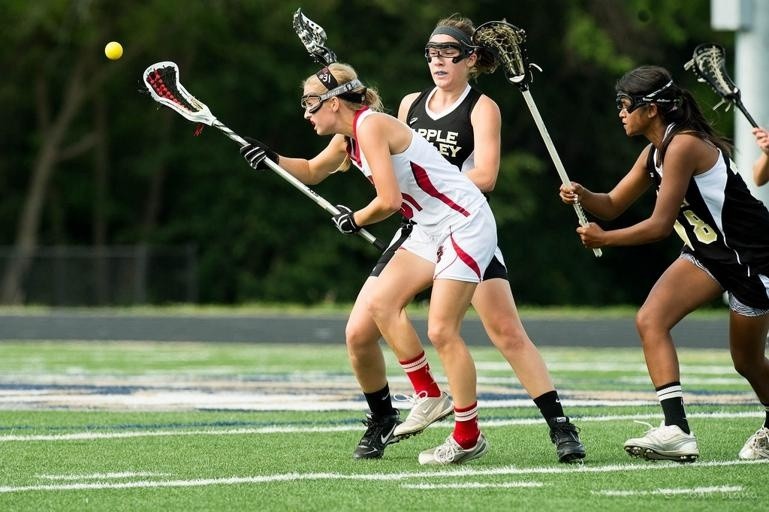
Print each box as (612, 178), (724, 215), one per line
(344, 19), (587, 463)
(749, 123), (768, 187)
(238, 62), (497, 468)
(558, 67), (769, 463)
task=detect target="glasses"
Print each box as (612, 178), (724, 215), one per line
(301, 79), (362, 114)
(616, 80), (674, 114)
(425, 40), (480, 65)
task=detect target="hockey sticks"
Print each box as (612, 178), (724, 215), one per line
(142, 61), (384, 251)
(291, 7), (338, 67)
(473, 19), (602, 259)
(685, 42), (759, 129)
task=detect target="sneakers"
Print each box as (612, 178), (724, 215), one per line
(550, 415), (586, 463)
(738, 420), (768, 461)
(622, 420), (699, 463)
(418, 429), (488, 465)
(393, 391), (454, 439)
(353, 410), (409, 461)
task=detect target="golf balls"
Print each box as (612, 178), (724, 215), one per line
(105, 41), (123, 60)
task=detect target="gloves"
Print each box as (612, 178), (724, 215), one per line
(239, 136), (278, 172)
(331, 204), (366, 238)
